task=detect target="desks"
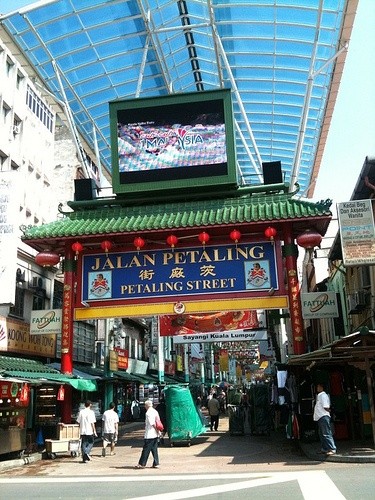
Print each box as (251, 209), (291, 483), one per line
(0, 427), (27, 455)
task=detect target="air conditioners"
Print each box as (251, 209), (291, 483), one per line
(347, 294), (359, 316)
(16, 268), (25, 280)
(34, 276), (46, 288)
(354, 290), (367, 306)
(13, 126), (18, 135)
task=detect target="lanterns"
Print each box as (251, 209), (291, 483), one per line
(167, 235), (177, 247)
(101, 240), (112, 252)
(297, 229), (322, 249)
(71, 242), (83, 254)
(134, 237), (144, 250)
(34, 252), (60, 267)
(198, 233), (211, 244)
(265, 227), (276, 240)
(230, 229), (240, 242)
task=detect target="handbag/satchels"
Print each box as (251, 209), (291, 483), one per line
(156, 415), (163, 431)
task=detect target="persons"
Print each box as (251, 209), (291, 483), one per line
(101, 402), (119, 457)
(155, 399), (167, 439)
(208, 393), (220, 431)
(194, 385), (248, 415)
(76, 399), (98, 463)
(134, 400), (161, 469)
(313, 382), (337, 455)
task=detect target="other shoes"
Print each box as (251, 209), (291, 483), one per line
(84, 452), (90, 461)
(135, 464), (145, 469)
(111, 452), (116, 455)
(326, 451), (333, 454)
(150, 464), (159, 469)
(317, 451), (326, 454)
(102, 448), (106, 457)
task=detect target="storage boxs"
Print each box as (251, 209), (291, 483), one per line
(45, 423), (82, 453)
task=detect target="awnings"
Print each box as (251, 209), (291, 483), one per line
(0, 363), (109, 391)
(112, 370), (178, 384)
